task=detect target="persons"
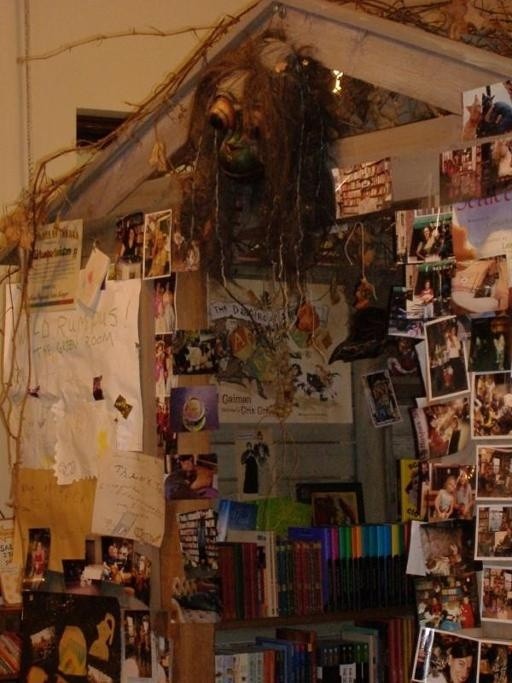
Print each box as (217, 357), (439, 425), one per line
(24, 539), (46, 586)
(353, 180), (512, 683)
(113, 211), (216, 497)
(103, 542), (131, 583)
(239, 433), (273, 496)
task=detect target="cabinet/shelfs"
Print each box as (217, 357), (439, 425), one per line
(168, 609), (419, 682)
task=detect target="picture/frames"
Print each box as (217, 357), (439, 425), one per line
(295, 482), (364, 527)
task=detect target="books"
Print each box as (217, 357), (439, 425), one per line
(75, 249), (109, 309)
(184, 452), (418, 683)
